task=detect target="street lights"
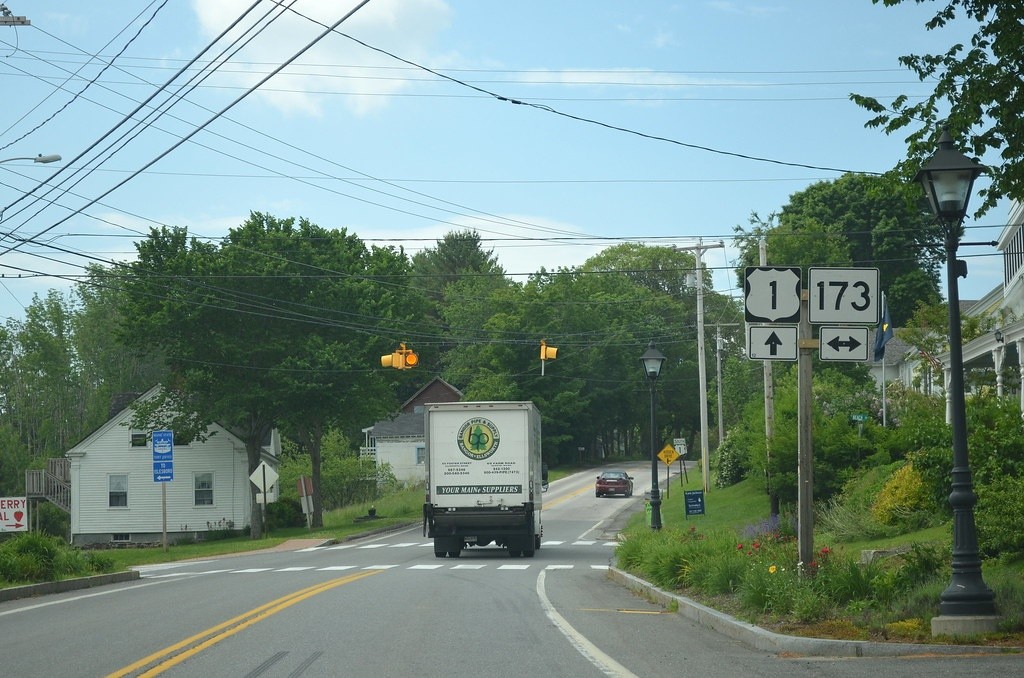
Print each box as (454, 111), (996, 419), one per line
(641, 338), (667, 530)
(908, 131), (1005, 639)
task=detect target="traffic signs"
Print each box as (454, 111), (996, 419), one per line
(153, 463), (174, 483)
(0, 497), (28, 532)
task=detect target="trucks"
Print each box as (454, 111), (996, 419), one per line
(423, 400), (549, 558)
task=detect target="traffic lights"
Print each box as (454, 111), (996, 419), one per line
(380, 351), (403, 369)
(404, 351), (421, 369)
(541, 340), (557, 362)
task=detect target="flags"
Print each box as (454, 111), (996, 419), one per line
(875, 304), (894, 362)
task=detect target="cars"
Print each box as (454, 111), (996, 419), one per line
(594, 469), (634, 498)
